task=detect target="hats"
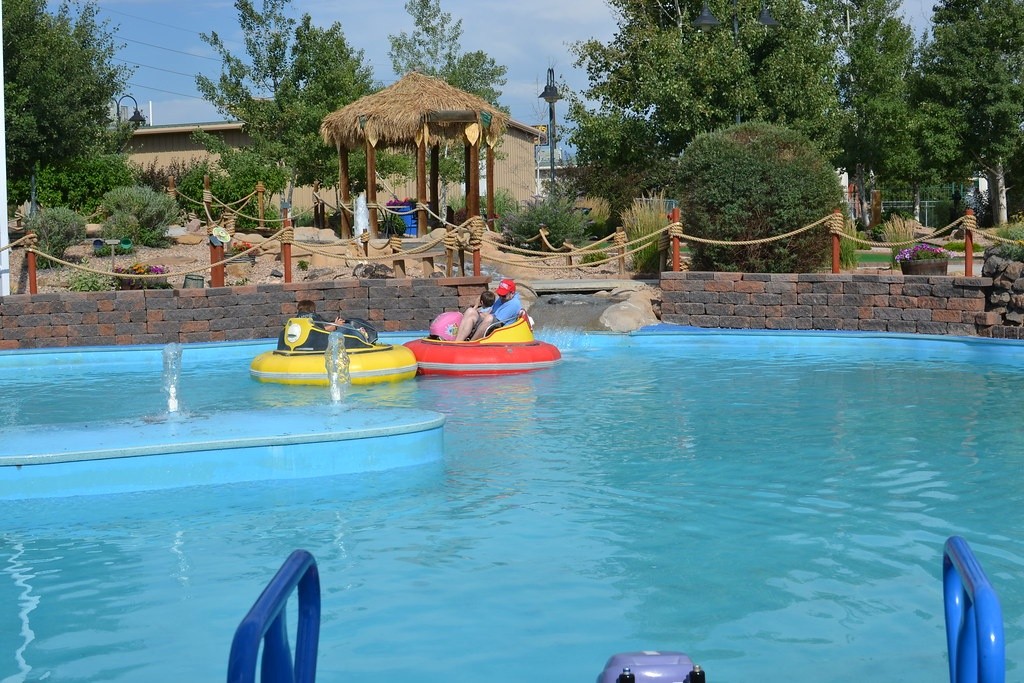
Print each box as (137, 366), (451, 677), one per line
(496, 279), (516, 296)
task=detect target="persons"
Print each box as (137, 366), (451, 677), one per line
(294, 300), (346, 332)
(455, 279), (522, 341)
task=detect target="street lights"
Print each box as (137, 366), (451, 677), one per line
(691, 0), (782, 125)
(97, 95), (146, 132)
(538, 68), (565, 195)
